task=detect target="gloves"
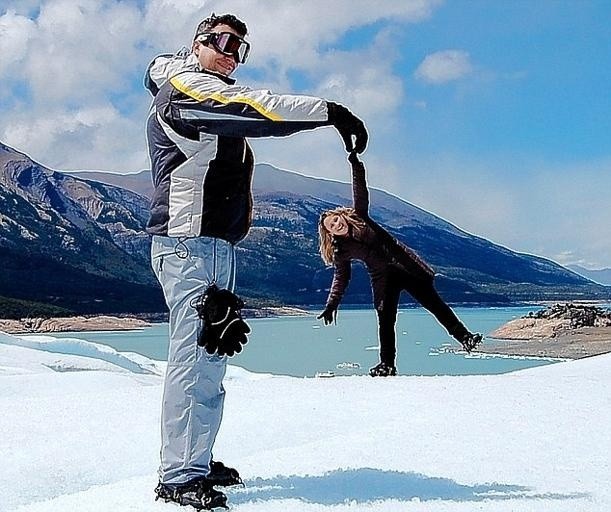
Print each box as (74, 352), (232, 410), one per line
(195, 283), (250, 357)
(317, 308), (333, 324)
(154, 477), (228, 509)
(325, 101), (368, 163)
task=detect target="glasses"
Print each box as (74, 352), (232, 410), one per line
(194, 32), (250, 65)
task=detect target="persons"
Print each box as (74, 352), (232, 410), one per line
(315, 150), (483, 374)
(140, 11), (369, 510)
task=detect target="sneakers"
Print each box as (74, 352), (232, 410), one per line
(207, 461), (239, 486)
(369, 362), (396, 376)
(463, 333), (482, 353)
